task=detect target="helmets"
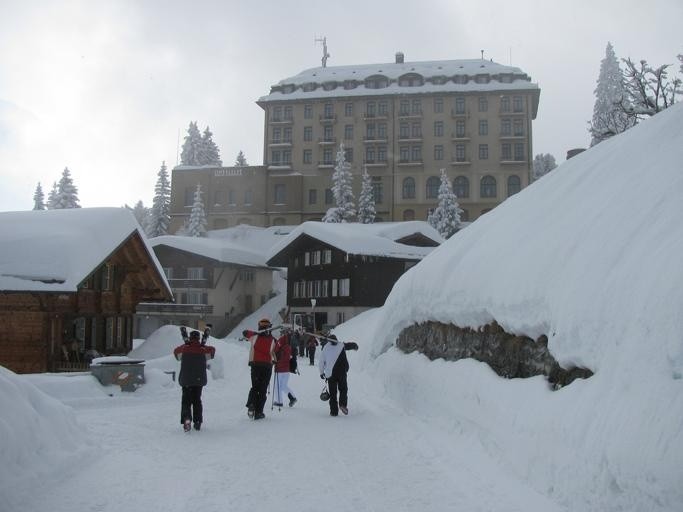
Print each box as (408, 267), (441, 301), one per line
(320, 392), (330, 401)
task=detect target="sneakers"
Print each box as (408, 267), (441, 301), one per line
(182, 414), (202, 431)
(248, 396), (299, 421)
(329, 408), (349, 417)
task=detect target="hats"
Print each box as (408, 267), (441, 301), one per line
(257, 318), (272, 328)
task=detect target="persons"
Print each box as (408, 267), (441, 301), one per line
(241, 318), (279, 420)
(271, 329), (298, 407)
(281, 327), (318, 365)
(317, 334), (358, 416)
(171, 331), (215, 432)
